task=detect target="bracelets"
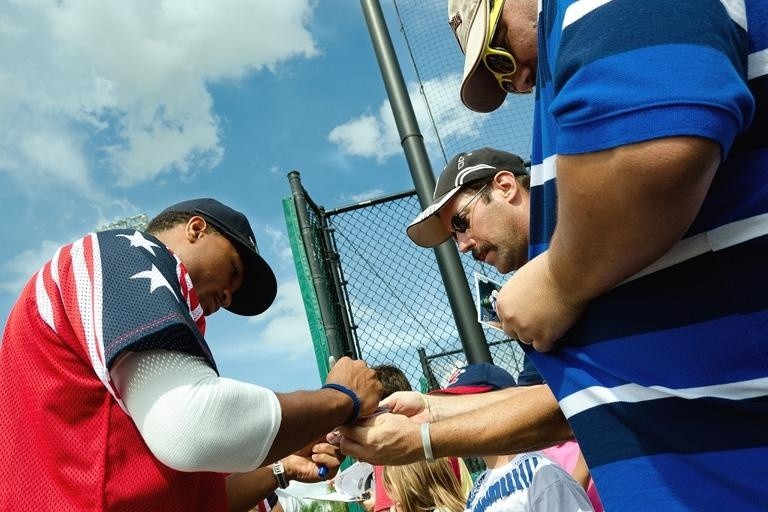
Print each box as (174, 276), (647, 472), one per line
(420, 422), (435, 461)
(271, 460), (289, 490)
(319, 382), (361, 426)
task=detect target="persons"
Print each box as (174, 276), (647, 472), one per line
(371, 364), (460, 511)
(380, 457), (467, 511)
(0, 197), (382, 510)
(443, 0), (768, 511)
(428, 361), (596, 511)
(337, 146), (572, 467)
(534, 439), (603, 511)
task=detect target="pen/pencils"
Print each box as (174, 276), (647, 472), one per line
(318, 466), (329, 477)
(328, 355), (338, 370)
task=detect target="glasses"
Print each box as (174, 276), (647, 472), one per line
(482, 0), (533, 96)
(451, 184), (489, 239)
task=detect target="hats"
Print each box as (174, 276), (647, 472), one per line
(406, 146), (528, 249)
(159, 197), (277, 317)
(447, 0), (508, 114)
(427, 361), (516, 395)
